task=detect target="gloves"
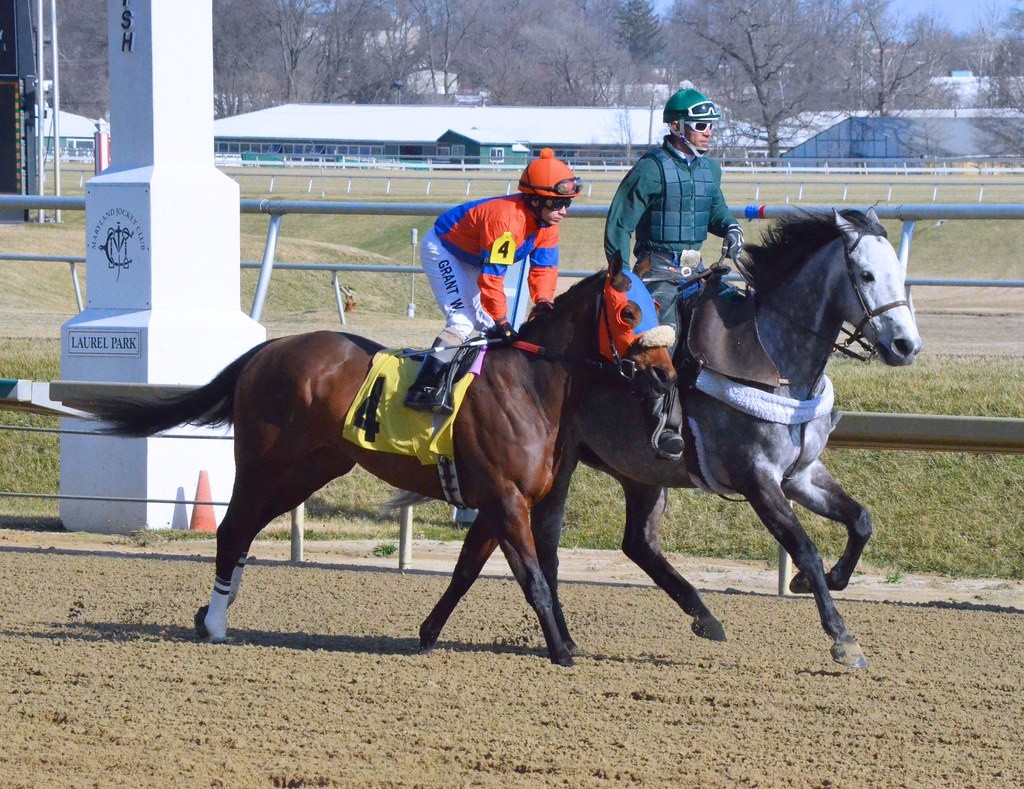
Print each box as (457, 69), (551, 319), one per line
(497, 322), (520, 348)
(721, 223), (745, 262)
(535, 302), (555, 313)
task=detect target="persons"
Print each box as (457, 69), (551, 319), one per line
(604, 81), (744, 461)
(403, 149), (583, 412)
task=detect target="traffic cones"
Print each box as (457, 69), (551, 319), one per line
(190, 470), (217, 532)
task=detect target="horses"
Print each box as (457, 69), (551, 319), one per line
(373, 202), (921, 668)
(75, 250), (677, 667)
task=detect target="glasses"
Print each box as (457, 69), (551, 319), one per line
(686, 101), (719, 116)
(679, 121), (713, 133)
(553, 176), (584, 195)
(536, 198), (572, 210)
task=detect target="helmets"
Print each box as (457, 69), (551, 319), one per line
(516, 147), (581, 198)
(663, 79), (721, 123)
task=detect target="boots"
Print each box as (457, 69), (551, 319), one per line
(649, 392), (685, 460)
(403, 352), (454, 416)
